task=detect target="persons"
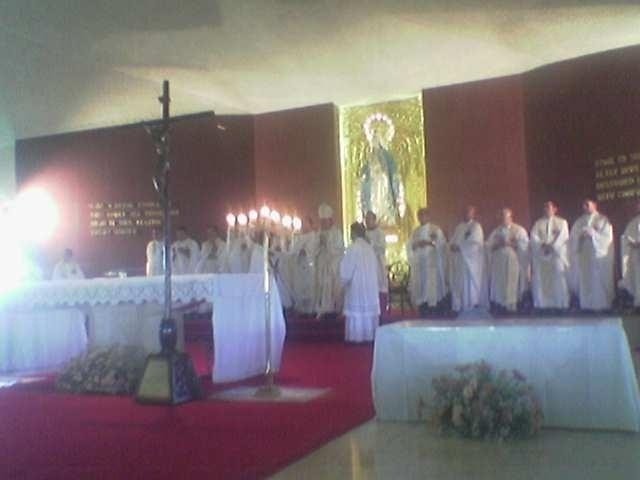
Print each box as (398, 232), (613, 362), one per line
(361, 210), (391, 326)
(485, 206), (535, 315)
(444, 204), (489, 321)
(619, 210), (640, 312)
(144, 201), (346, 331)
(335, 220), (383, 348)
(526, 196), (576, 319)
(565, 192), (618, 317)
(51, 247), (86, 282)
(403, 205), (452, 320)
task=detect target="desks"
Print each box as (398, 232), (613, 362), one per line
(373, 316), (640, 432)
(0, 274), (285, 384)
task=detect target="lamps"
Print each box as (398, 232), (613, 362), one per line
(227, 206), (304, 397)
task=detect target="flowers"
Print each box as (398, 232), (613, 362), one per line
(56, 340), (155, 395)
(416, 357), (544, 441)
